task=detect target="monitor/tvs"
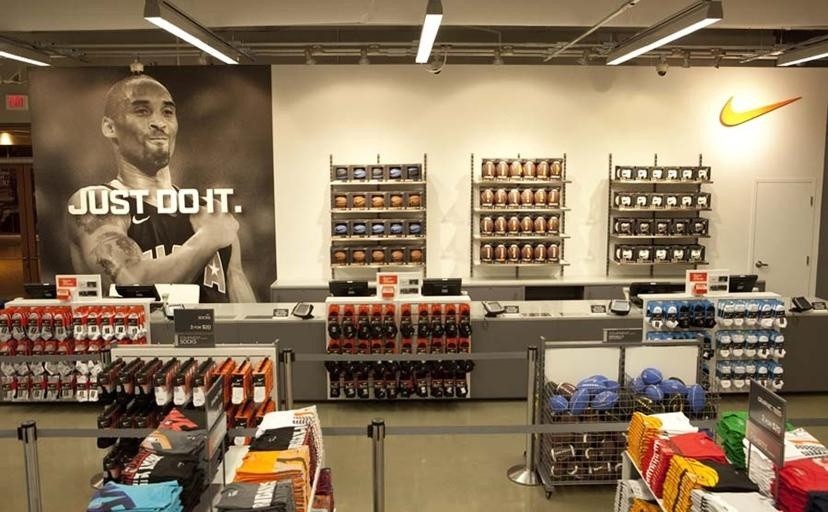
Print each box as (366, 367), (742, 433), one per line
(329, 280), (370, 296)
(116, 283), (159, 298)
(25, 282), (54, 297)
(423, 278), (461, 295)
(729, 275), (757, 293)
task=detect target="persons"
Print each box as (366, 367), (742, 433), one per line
(64, 74), (258, 302)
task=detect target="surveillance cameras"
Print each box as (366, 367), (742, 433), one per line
(131, 63), (144, 75)
(657, 63), (669, 76)
(432, 61), (443, 74)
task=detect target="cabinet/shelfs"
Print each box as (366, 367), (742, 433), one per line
(715, 296), (784, 395)
(398, 299), (473, 399)
(0, 156), (42, 304)
(645, 296), (715, 395)
(326, 300), (398, 401)
(77, 302), (149, 403)
(326, 150), (431, 276)
(3, 305), (76, 409)
(604, 153), (716, 277)
(468, 151), (571, 277)
(616, 423), (828, 510)
(97, 343), (278, 451)
(93, 444), (333, 511)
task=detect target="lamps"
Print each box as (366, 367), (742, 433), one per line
(1, 36), (60, 72)
(777, 33), (827, 69)
(604, 1), (725, 68)
(414, 1), (447, 66)
(142, 1), (257, 69)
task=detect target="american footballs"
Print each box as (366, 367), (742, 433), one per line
(542, 369), (715, 478)
(481, 188), (560, 204)
(335, 225), (421, 233)
(480, 216), (559, 232)
(335, 250), (422, 261)
(335, 196), (419, 207)
(337, 168), (418, 178)
(480, 244), (559, 259)
(483, 161), (561, 176)
